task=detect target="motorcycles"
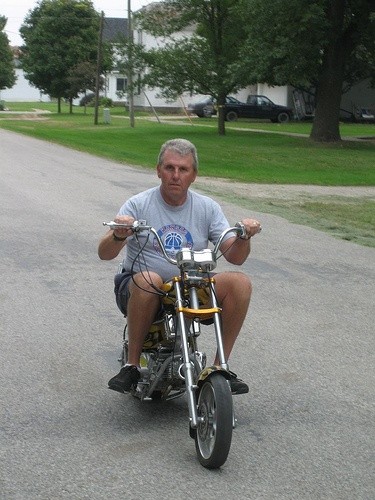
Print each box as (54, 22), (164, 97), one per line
(100, 219), (263, 470)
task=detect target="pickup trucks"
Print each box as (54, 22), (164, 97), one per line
(224, 94), (294, 123)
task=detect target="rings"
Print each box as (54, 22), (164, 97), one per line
(253, 222), (257, 225)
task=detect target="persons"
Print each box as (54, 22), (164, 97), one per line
(97, 138), (262, 395)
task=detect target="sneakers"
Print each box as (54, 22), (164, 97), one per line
(228, 370), (249, 394)
(108, 366), (141, 391)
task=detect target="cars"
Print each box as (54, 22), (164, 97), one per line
(187, 95), (242, 119)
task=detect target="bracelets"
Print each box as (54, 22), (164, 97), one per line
(237, 235), (250, 240)
(113, 232), (127, 241)
